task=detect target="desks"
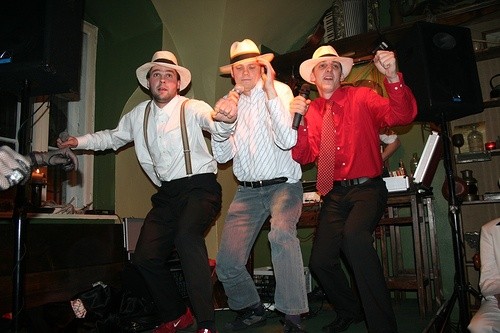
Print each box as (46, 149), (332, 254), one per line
(245, 191), (442, 321)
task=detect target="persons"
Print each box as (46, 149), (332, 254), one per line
(57, 50), (238, 333)
(211, 38), (310, 333)
(289, 45), (417, 333)
(379, 133), (401, 168)
(0, 145), (78, 190)
(468, 217), (500, 333)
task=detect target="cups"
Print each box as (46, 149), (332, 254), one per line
(463, 192), (477, 202)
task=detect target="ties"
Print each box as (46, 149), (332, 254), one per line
(316, 100), (335, 196)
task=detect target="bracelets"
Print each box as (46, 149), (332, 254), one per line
(29, 151), (40, 167)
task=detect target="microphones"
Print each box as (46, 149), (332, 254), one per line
(292, 84), (311, 131)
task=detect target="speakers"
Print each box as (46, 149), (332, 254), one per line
(393, 22), (484, 123)
(0, 0), (85, 102)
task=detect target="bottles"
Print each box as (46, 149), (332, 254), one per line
(478, 191), (500, 201)
(467, 126), (484, 152)
(399, 152), (420, 175)
(460, 169), (477, 194)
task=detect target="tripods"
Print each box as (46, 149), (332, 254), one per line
(421, 122), (482, 333)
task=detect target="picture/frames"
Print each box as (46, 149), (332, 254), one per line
(481, 28), (500, 48)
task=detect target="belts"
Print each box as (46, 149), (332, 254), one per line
(237, 177), (288, 190)
(332, 177), (367, 185)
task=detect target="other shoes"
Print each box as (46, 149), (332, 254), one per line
(195, 320), (217, 333)
(320, 312), (352, 333)
(280, 314), (304, 333)
(231, 305), (267, 331)
(152, 306), (194, 333)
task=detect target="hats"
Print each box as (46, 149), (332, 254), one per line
(219, 39), (274, 74)
(136, 51), (191, 91)
(299, 46), (354, 85)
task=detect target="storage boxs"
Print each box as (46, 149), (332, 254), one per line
(252, 266), (312, 302)
(381, 130), (443, 194)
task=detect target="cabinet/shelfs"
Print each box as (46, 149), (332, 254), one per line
(428, 0), (500, 319)
